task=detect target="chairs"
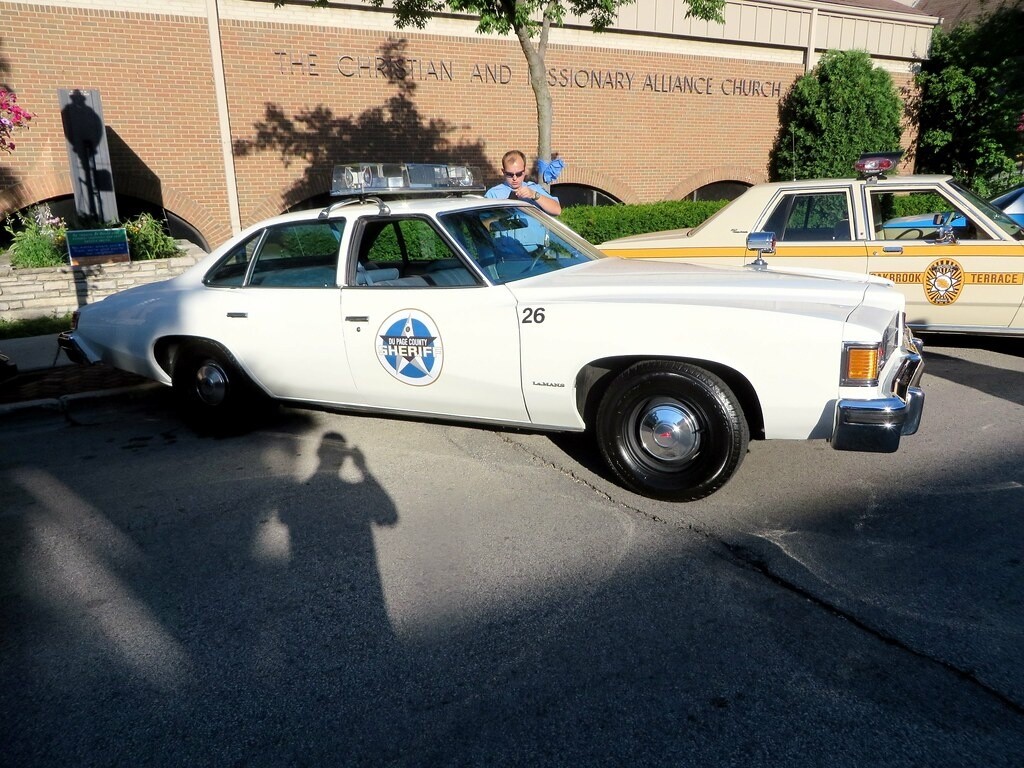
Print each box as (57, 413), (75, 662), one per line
(421, 258), (478, 286)
(356, 268), (430, 286)
(833, 219), (850, 241)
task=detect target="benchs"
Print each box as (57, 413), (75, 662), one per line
(253, 265), (336, 288)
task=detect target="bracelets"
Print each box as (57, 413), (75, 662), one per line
(533, 191), (540, 201)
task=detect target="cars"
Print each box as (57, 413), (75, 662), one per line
(58, 160), (926, 502)
(882, 183), (1024, 239)
(593, 157), (1024, 337)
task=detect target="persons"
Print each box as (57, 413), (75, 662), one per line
(477, 151), (561, 259)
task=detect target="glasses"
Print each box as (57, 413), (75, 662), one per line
(503, 165), (525, 178)
